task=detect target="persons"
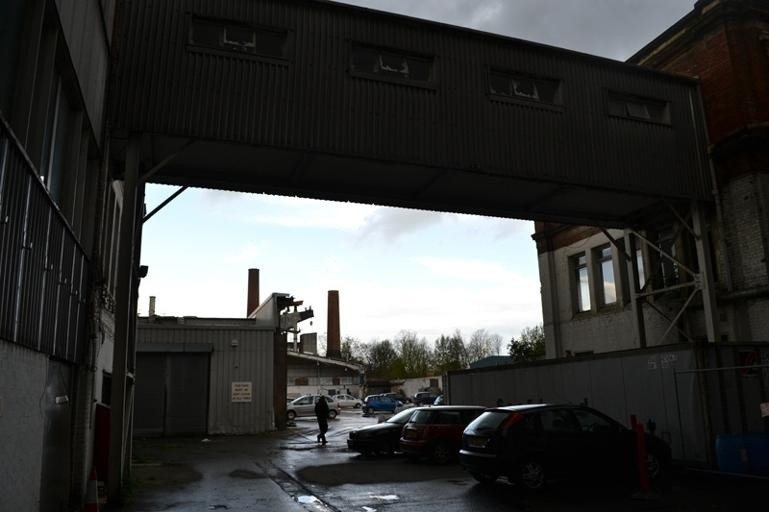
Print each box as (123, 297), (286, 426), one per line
(314, 395), (330, 443)
